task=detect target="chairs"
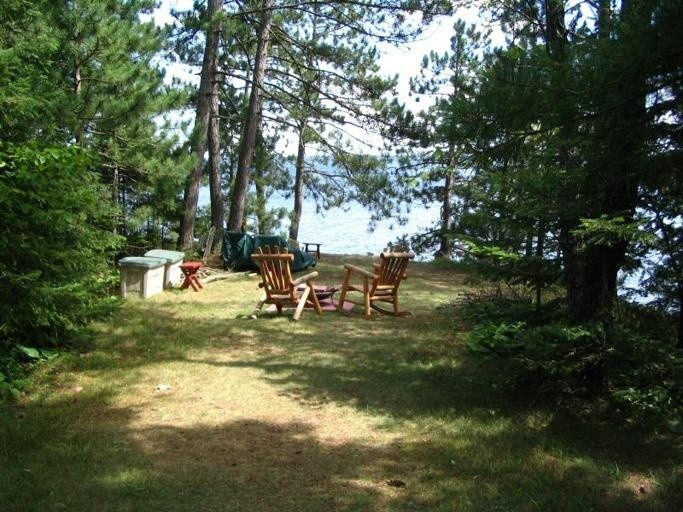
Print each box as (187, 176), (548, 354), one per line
(337, 250), (416, 322)
(250, 244), (322, 323)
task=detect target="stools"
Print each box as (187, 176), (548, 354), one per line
(302, 243), (323, 259)
(178, 261), (204, 292)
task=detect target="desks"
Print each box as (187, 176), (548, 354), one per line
(297, 284), (339, 305)
